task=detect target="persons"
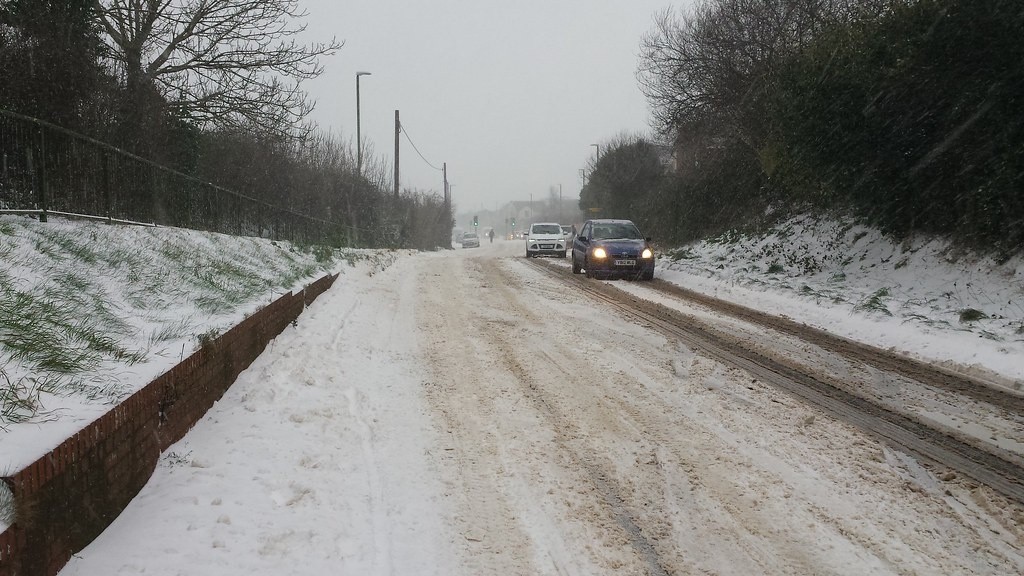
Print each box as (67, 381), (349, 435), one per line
(572, 225), (576, 243)
(618, 227), (628, 238)
(489, 229), (495, 243)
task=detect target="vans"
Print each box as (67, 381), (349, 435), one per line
(561, 225), (573, 244)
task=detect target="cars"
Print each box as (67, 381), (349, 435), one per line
(570, 217), (655, 280)
(462, 233), (480, 248)
(524, 223), (569, 259)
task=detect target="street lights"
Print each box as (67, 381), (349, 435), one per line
(578, 169), (585, 187)
(357, 70), (372, 178)
(557, 184), (562, 219)
(590, 145), (599, 169)
(449, 185), (456, 212)
(529, 193), (533, 222)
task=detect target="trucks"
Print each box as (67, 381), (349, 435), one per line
(481, 226), (495, 239)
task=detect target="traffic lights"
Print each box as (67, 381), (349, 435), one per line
(512, 221), (515, 226)
(474, 216), (478, 226)
